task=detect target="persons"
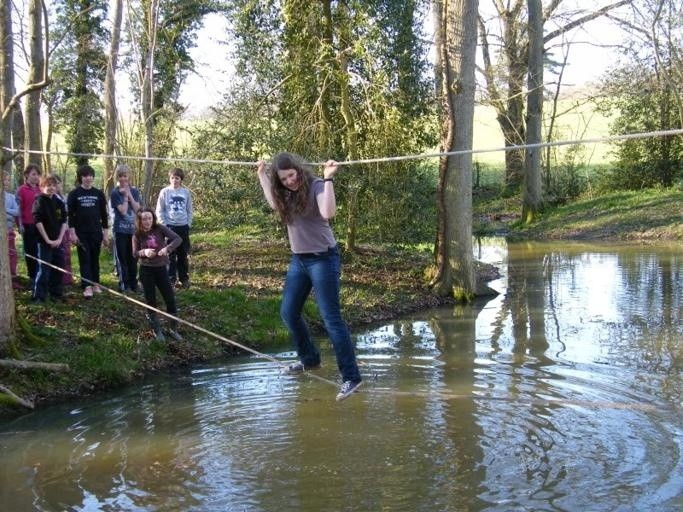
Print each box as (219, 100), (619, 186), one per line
(109, 165), (143, 295)
(154, 168), (192, 288)
(29, 176), (66, 306)
(255, 151), (365, 402)
(131, 207), (183, 344)
(52, 173), (73, 284)
(15, 164), (43, 290)
(3, 170), (21, 291)
(67, 165), (108, 299)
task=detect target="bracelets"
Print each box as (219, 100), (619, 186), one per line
(123, 200), (129, 202)
(324, 178), (334, 182)
(17, 223), (22, 226)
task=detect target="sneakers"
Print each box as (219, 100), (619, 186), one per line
(170, 277), (190, 292)
(83, 285), (103, 297)
(153, 328), (183, 345)
(336, 379), (364, 402)
(119, 286), (143, 297)
(284, 360), (322, 375)
